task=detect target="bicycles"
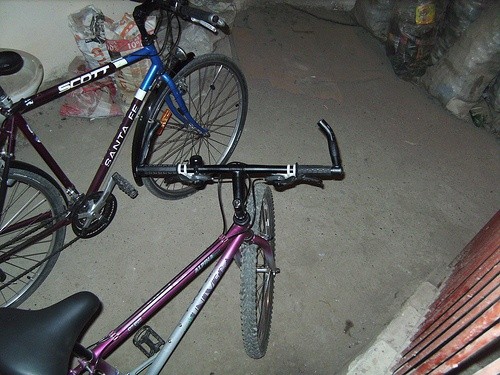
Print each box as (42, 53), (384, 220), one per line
(0, 0), (251, 312)
(1, 116), (346, 375)
